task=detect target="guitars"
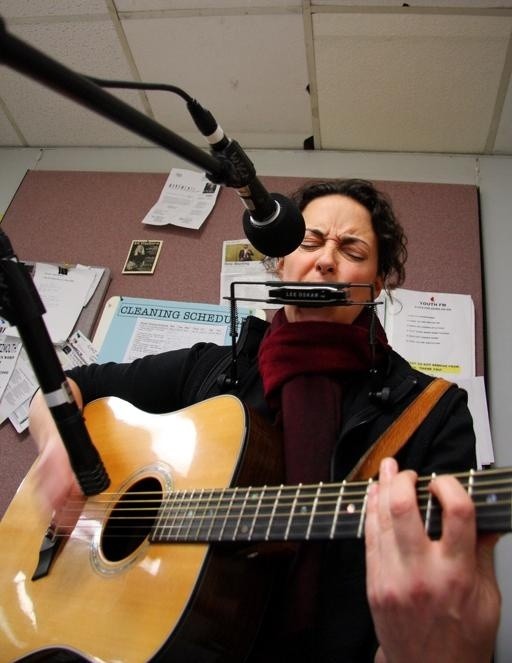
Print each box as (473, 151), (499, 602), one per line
(0, 396), (511, 663)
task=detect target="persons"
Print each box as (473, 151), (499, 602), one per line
(239, 244), (254, 261)
(23, 180), (502, 662)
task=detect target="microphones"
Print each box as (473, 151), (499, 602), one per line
(186, 97), (306, 258)
